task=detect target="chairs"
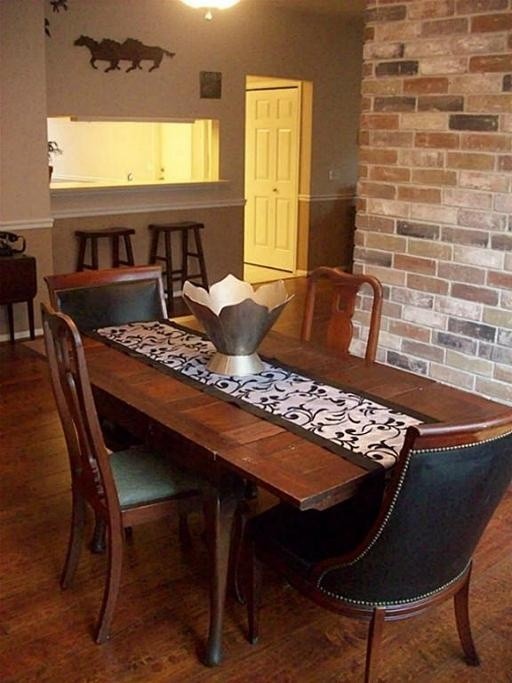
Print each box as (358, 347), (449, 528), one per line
(300, 268), (382, 361)
(44, 265), (207, 535)
(39, 300), (250, 646)
(243, 411), (512, 682)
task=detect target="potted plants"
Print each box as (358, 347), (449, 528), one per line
(48, 138), (61, 186)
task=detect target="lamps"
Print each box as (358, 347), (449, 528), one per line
(182, 0), (240, 19)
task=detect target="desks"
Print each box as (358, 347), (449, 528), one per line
(1, 250), (37, 344)
(21, 314), (512, 667)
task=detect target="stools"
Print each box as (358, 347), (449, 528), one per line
(74, 226), (136, 271)
(149, 221), (209, 312)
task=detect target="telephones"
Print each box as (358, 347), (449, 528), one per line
(0, 231), (18, 257)
